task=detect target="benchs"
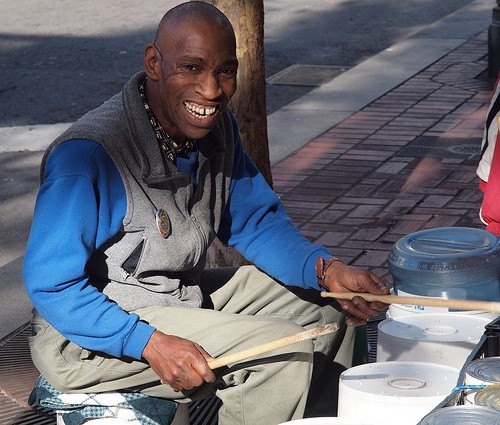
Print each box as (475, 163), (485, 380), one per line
(0, 317), (224, 425)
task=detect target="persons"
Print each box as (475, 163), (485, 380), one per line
(476, 76), (499, 240)
(23, 0), (392, 425)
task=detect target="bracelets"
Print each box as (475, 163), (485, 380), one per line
(316, 256), (343, 291)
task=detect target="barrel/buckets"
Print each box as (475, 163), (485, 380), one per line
(387, 227), (500, 302)
(269, 289), (500, 425)
